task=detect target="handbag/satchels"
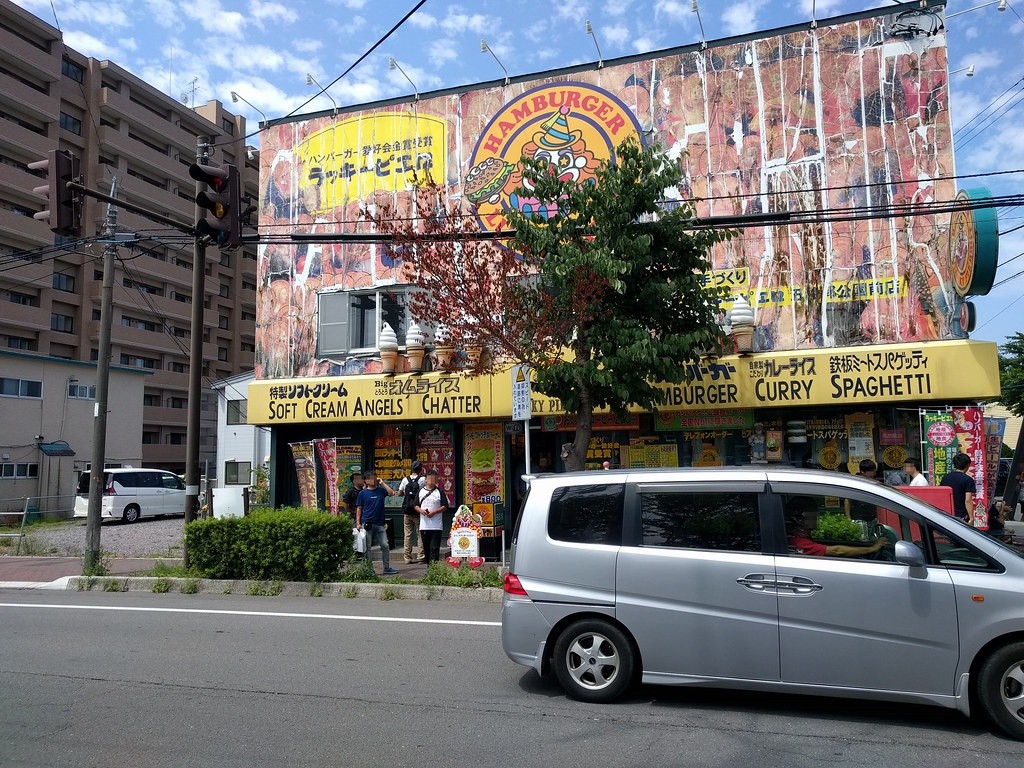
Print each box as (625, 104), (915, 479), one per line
(352, 528), (367, 553)
(852, 520), (868, 541)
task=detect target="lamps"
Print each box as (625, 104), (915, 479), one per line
(586, 20), (604, 68)
(480, 39), (510, 85)
(306, 72), (338, 114)
(691, 0), (707, 50)
(247, 149), (259, 160)
(389, 57), (420, 100)
(946, 0), (1006, 19)
(949, 64), (975, 76)
(231, 91), (268, 127)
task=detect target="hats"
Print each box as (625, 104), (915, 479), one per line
(411, 461), (422, 472)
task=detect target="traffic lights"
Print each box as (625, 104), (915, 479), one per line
(28, 150), (76, 236)
(189, 163), (240, 255)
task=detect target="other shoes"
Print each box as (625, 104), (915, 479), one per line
(418, 560), (426, 564)
(405, 561), (411, 563)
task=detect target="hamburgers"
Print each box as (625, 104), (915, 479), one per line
(470, 439), (495, 499)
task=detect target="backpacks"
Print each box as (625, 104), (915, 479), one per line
(402, 475), (422, 515)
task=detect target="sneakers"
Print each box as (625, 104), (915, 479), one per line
(367, 570), (375, 576)
(383, 568), (398, 573)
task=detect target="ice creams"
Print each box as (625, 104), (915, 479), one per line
(731, 294), (753, 349)
(378, 322), (398, 371)
(435, 323), (482, 366)
(405, 322), (425, 369)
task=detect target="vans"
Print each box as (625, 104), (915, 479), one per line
(501, 462), (1024, 743)
(73, 468), (200, 525)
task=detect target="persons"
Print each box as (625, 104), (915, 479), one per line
(846, 459), (881, 542)
(355, 470), (398, 576)
(901, 454), (928, 487)
(398, 461), (425, 564)
(1015, 456), (1024, 522)
(412, 468), (449, 564)
(938, 452), (978, 526)
(781, 504), (890, 559)
(344, 472), (365, 522)
